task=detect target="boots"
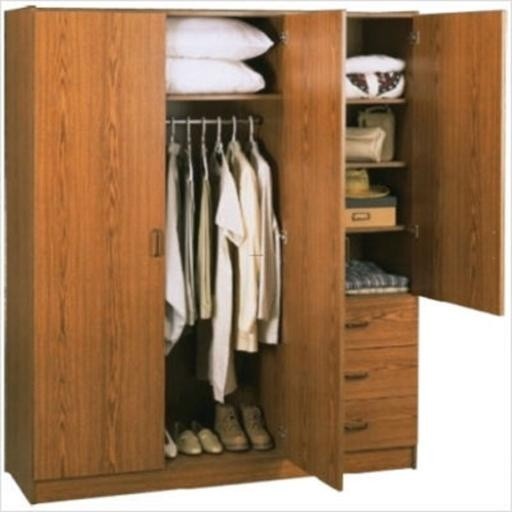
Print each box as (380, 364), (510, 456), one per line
(241, 404), (273, 450)
(214, 405), (249, 451)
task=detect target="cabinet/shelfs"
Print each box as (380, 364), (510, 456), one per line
(343, 294), (418, 472)
(343, 8), (506, 320)
(3, 3), (343, 507)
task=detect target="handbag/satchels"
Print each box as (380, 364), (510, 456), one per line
(345, 107), (396, 163)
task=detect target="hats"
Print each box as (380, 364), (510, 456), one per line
(346, 169), (390, 199)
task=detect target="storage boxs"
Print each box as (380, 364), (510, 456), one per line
(342, 193), (397, 224)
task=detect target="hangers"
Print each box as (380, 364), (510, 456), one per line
(165, 114), (272, 181)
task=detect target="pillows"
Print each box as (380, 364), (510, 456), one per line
(166, 56), (267, 93)
(164, 16), (276, 58)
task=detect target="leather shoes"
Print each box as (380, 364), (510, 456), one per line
(175, 422), (202, 455)
(192, 420), (223, 454)
